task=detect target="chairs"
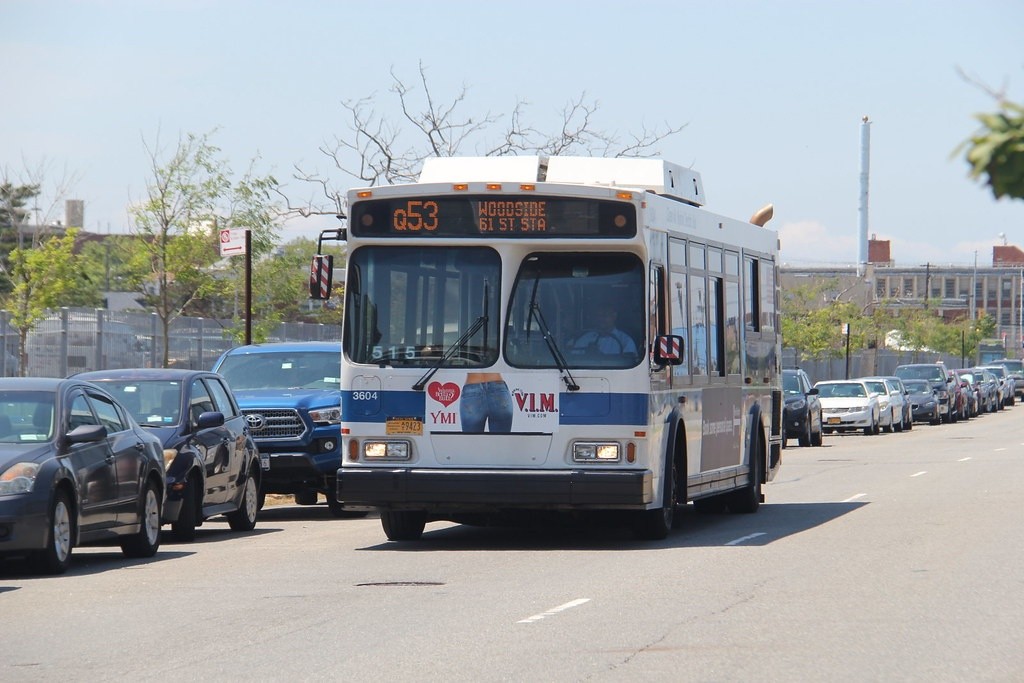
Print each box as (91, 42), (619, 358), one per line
(33, 404), (54, 434)
(152, 389), (182, 420)
(116, 391), (140, 419)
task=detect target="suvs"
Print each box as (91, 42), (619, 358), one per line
(213, 343), (341, 511)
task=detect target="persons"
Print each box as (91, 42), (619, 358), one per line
(569, 300), (639, 358)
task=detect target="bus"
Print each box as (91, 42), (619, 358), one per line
(307, 156), (784, 542)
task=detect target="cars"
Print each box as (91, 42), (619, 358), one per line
(782, 368), (824, 447)
(67, 369), (266, 537)
(813, 376), (913, 435)
(895, 360), (1024, 425)
(0, 376), (166, 575)
